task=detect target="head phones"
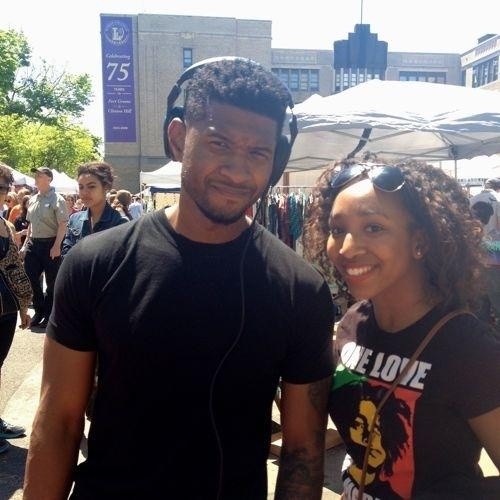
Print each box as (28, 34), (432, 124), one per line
(163, 56), (298, 187)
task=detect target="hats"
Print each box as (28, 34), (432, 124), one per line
(31, 167), (53, 182)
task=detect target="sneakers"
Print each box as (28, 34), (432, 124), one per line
(0, 437), (11, 453)
(0, 418), (25, 438)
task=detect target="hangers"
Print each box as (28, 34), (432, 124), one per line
(262, 183), (322, 203)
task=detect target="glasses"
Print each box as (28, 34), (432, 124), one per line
(330, 162), (413, 200)
(0, 186), (11, 193)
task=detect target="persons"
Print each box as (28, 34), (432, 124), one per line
(21, 59), (334, 500)
(0, 161), (147, 455)
(326, 379), (411, 500)
(469, 178), (500, 321)
(301, 158), (500, 500)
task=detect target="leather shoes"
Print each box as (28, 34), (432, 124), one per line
(30, 313), (44, 323)
(40, 319), (48, 328)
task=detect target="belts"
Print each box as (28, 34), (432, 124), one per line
(31, 238), (51, 241)
